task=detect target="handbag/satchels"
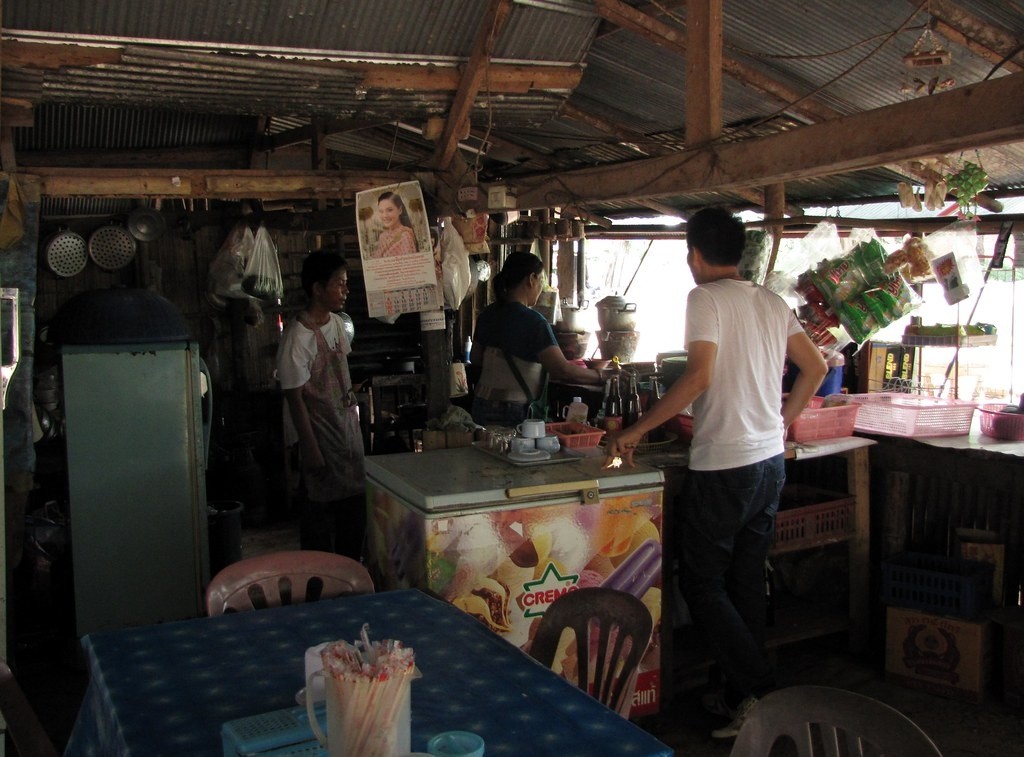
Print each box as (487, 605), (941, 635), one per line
(208, 221), (284, 304)
(524, 395), (566, 422)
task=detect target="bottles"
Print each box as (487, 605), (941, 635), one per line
(271, 314), (283, 339)
(562, 371), (666, 444)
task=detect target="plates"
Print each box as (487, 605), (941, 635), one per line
(508, 449), (550, 462)
(296, 687), (309, 705)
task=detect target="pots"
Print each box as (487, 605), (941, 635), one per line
(595, 291), (638, 331)
(554, 296), (590, 334)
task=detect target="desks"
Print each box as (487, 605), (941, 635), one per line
(62, 589), (677, 757)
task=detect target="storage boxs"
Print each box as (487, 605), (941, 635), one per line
(773, 484), (1005, 706)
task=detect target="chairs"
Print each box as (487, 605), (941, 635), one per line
(518, 588), (653, 721)
(730, 684), (944, 757)
(0, 659), (57, 757)
(206, 551), (375, 617)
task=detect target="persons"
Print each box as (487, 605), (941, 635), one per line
(470, 251), (614, 429)
(600, 207), (828, 738)
(375, 192), (419, 258)
(276, 251), (365, 562)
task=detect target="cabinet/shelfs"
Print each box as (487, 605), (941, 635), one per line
(901, 255), (1016, 409)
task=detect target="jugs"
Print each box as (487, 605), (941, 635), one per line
(305, 663), (422, 756)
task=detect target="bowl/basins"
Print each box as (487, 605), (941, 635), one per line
(583, 358), (612, 370)
(352, 383), (361, 392)
(345, 285), (358, 303)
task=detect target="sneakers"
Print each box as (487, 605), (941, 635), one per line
(711, 697), (759, 739)
(702, 690), (734, 718)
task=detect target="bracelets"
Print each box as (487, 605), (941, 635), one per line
(596, 368), (602, 383)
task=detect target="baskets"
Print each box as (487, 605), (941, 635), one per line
(976, 402), (1024, 442)
(843, 392), (979, 437)
(675, 412), (694, 442)
(545, 422), (606, 448)
(635, 432), (678, 454)
(773, 482), (857, 553)
(781, 390), (860, 444)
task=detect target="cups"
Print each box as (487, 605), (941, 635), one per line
(310, 235), (322, 251)
(318, 624), (416, 683)
(504, 218), (584, 239)
(401, 361), (415, 374)
(476, 419), (561, 455)
(410, 731), (487, 757)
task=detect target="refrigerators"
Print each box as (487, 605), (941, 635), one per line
(363, 446), (665, 716)
(61, 338), (212, 636)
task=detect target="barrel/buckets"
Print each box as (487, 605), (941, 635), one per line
(786, 349), (846, 397)
(208, 499), (244, 581)
